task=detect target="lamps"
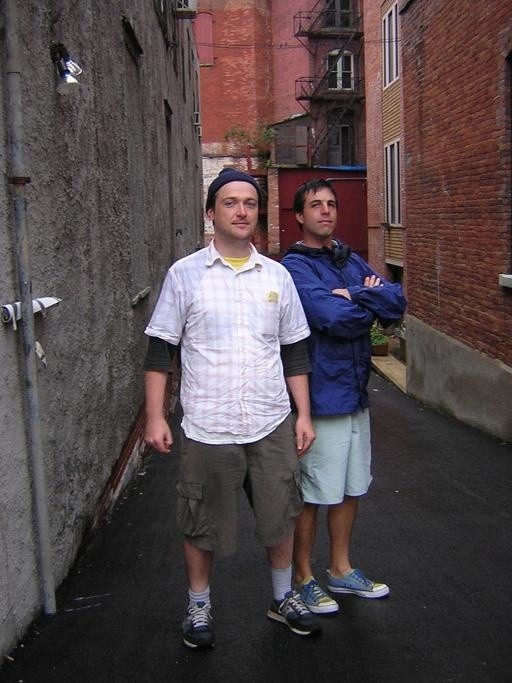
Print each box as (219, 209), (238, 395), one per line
(48, 40), (83, 95)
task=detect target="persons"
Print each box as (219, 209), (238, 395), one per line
(280, 179), (408, 613)
(143, 168), (321, 649)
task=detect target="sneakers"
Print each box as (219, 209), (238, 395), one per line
(181, 603), (213, 649)
(266, 568), (389, 636)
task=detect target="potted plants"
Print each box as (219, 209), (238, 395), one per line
(370, 327), (389, 356)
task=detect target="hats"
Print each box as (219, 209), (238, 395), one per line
(206, 168), (261, 212)
